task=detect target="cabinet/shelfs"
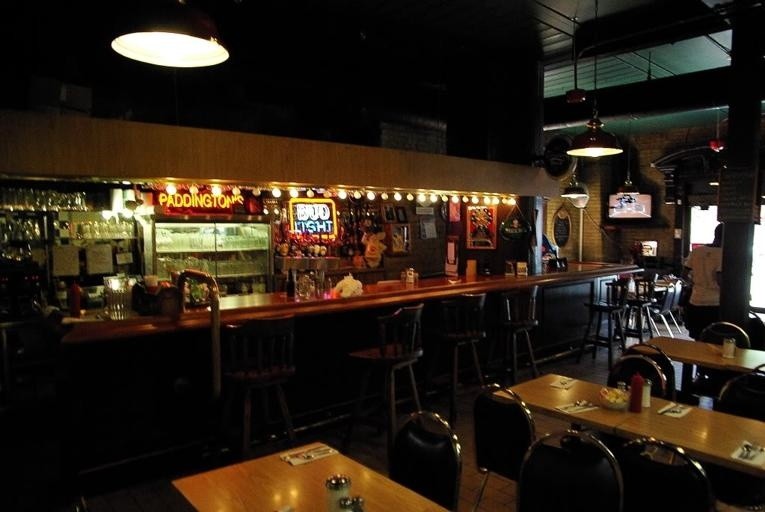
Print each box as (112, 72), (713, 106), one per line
(133, 203), (272, 296)
(0, 208), (147, 308)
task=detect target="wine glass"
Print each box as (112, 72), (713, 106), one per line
(0, 188), (88, 216)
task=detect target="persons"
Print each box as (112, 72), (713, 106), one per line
(682, 223), (730, 342)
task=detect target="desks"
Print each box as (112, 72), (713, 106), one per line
(631, 336), (765, 380)
(491, 373), (765, 482)
(170, 441), (452, 512)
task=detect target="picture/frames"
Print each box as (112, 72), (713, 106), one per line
(466, 206), (497, 250)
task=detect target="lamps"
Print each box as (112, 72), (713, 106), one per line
(616, 119), (641, 196)
(111, 0), (231, 70)
(566, 15), (587, 103)
(566, 0), (625, 161)
(561, 173), (588, 200)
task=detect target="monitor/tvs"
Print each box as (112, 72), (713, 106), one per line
(607, 192), (654, 220)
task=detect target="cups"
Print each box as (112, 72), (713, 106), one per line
(103, 276), (131, 319)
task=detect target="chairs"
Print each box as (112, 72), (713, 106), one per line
(607, 343), (765, 423)
(210, 316), (299, 463)
(486, 284), (542, 386)
(419, 291), (487, 430)
(341, 302), (425, 455)
(579, 271), (682, 370)
(690, 320), (752, 397)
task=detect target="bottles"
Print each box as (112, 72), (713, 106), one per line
(642, 378), (652, 406)
(0, 217), (40, 262)
(56, 281), (68, 310)
(70, 279), (81, 315)
(618, 382), (626, 390)
(285, 267), (295, 298)
(629, 374), (645, 414)
(332, 203), (381, 257)
(324, 472), (366, 512)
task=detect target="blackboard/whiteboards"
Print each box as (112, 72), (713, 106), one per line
(553, 212), (571, 249)
(718, 163), (759, 223)
